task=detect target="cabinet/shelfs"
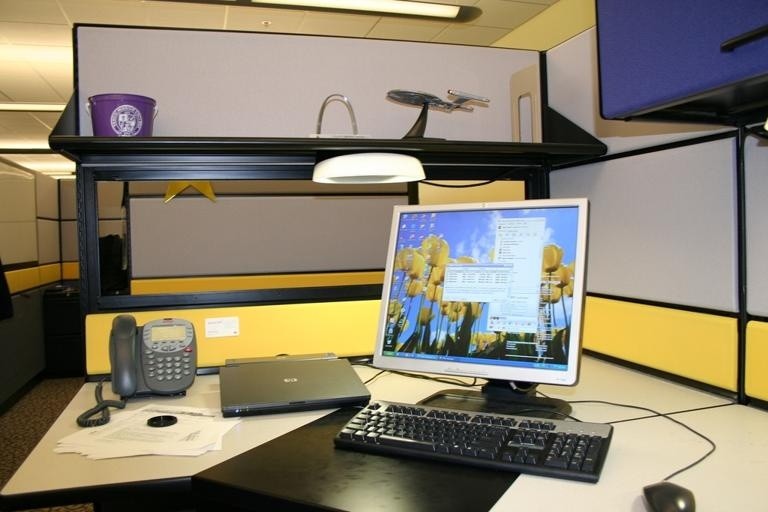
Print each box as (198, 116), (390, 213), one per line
(49, 24), (608, 382)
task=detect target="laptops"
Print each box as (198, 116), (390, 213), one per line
(219, 353), (372, 418)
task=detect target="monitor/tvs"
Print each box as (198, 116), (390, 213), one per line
(372, 197), (590, 419)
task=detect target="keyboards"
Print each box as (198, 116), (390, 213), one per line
(333, 399), (614, 483)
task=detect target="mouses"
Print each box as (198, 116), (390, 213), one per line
(642, 483), (697, 511)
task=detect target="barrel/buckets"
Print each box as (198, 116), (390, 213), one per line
(84, 93), (158, 137)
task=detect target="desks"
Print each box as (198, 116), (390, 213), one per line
(0, 350), (768, 512)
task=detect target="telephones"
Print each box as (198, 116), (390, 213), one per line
(110, 315), (196, 400)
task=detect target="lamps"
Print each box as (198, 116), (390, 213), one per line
(311, 94), (426, 185)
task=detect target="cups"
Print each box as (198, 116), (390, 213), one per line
(90, 94), (156, 138)
